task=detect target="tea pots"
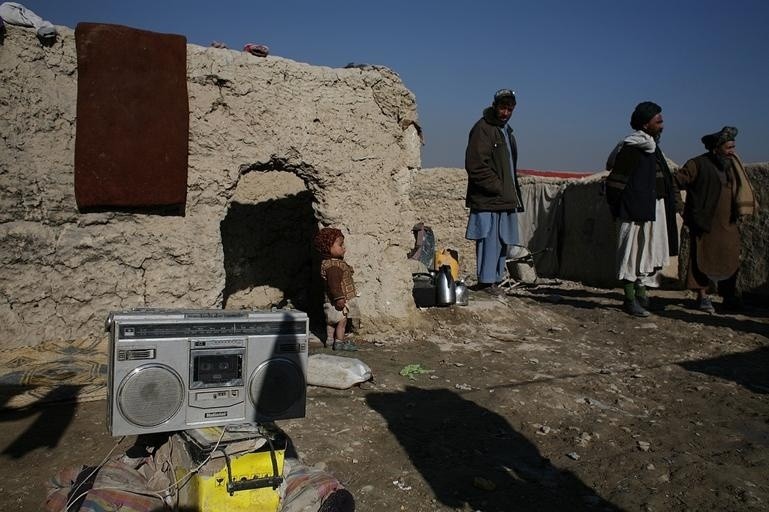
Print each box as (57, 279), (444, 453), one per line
(412, 265), (456, 306)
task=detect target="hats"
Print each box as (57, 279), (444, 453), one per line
(700, 125), (740, 151)
(312, 226), (344, 260)
(629, 101), (664, 132)
(493, 88), (518, 105)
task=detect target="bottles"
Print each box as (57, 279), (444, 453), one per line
(455, 282), (468, 305)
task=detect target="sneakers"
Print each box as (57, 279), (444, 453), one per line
(637, 295), (666, 311)
(722, 295), (746, 312)
(695, 296), (716, 314)
(478, 281), (508, 298)
(333, 339), (360, 352)
(325, 337), (334, 348)
(619, 300), (651, 317)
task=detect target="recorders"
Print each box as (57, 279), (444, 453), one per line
(105, 306), (309, 437)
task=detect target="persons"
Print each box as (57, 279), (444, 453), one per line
(670, 125), (759, 312)
(315, 227), (358, 351)
(463, 88), (523, 299)
(604, 99), (680, 320)
